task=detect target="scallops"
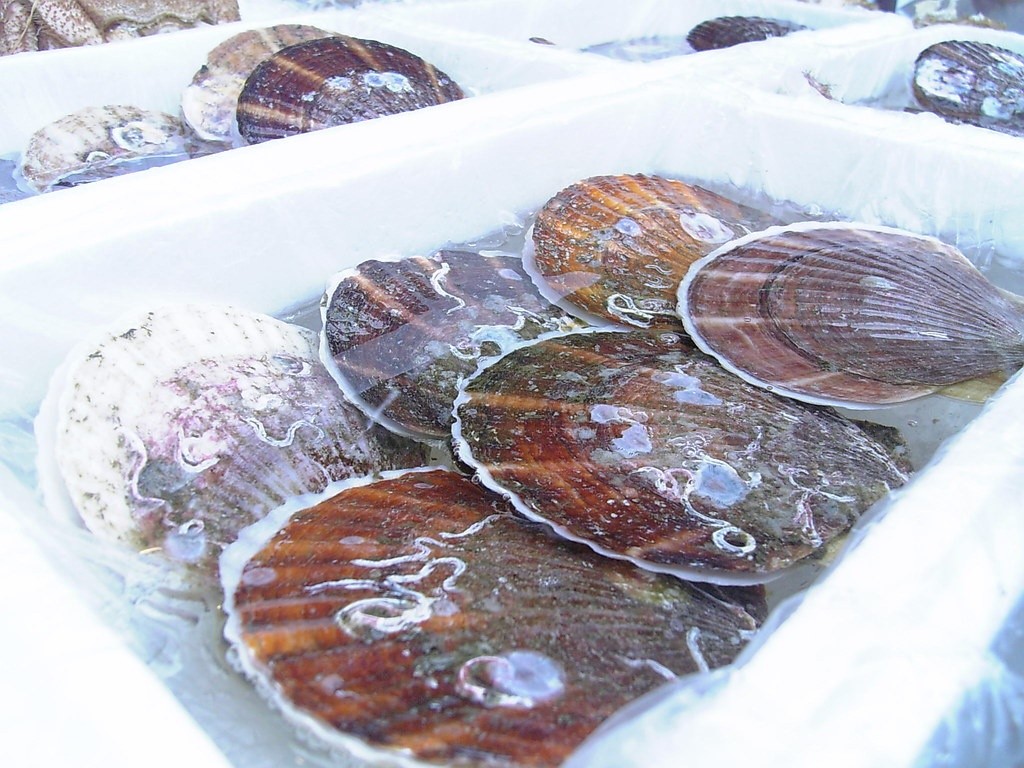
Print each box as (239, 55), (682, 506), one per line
(527, 0), (1024, 140)
(0, 0), (466, 196)
(30, 171), (1023, 768)
